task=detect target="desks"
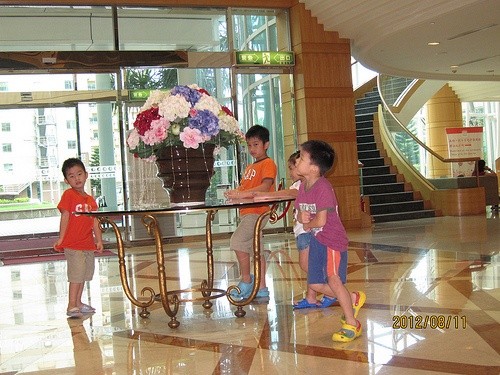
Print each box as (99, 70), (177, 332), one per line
(72, 197), (296, 330)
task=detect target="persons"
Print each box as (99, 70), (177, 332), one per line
(54, 158), (102, 319)
(225, 124), (277, 302)
(294, 140), (366, 342)
(472, 160), (500, 209)
(252, 150), (336, 308)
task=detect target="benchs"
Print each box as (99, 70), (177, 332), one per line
(426, 175), (500, 213)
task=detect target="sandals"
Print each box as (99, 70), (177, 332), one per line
(340, 291), (366, 324)
(67, 307), (83, 317)
(67, 312), (94, 327)
(78, 303), (96, 312)
(231, 274), (255, 297)
(332, 320), (362, 341)
(242, 287), (269, 302)
(332, 336), (365, 354)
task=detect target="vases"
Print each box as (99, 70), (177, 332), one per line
(153, 143), (217, 207)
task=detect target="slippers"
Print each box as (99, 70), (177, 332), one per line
(318, 296), (336, 308)
(293, 298), (317, 309)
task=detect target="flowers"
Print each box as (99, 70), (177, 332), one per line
(126, 84), (246, 159)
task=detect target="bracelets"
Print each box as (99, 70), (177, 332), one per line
(488, 169), (491, 171)
